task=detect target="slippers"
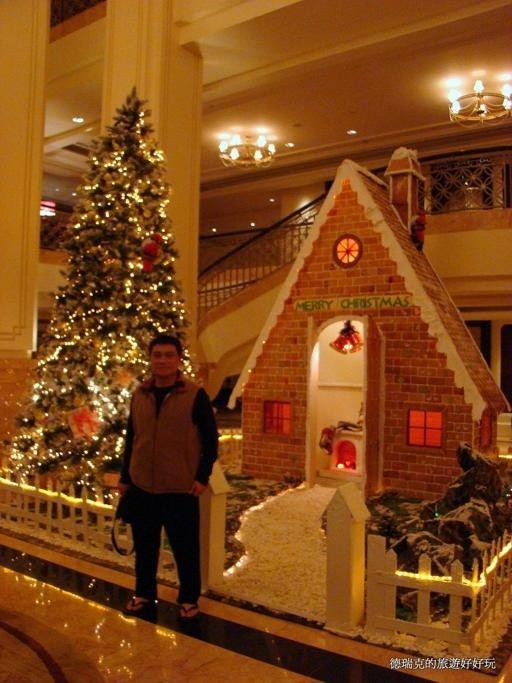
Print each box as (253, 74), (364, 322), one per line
(124, 599), (152, 615)
(179, 606), (199, 618)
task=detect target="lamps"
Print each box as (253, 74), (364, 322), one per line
(448, 77), (512, 128)
(217, 134), (277, 169)
(329, 319), (363, 355)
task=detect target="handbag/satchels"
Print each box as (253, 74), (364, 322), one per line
(116, 495), (132, 523)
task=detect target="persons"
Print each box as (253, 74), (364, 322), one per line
(118, 336), (218, 621)
